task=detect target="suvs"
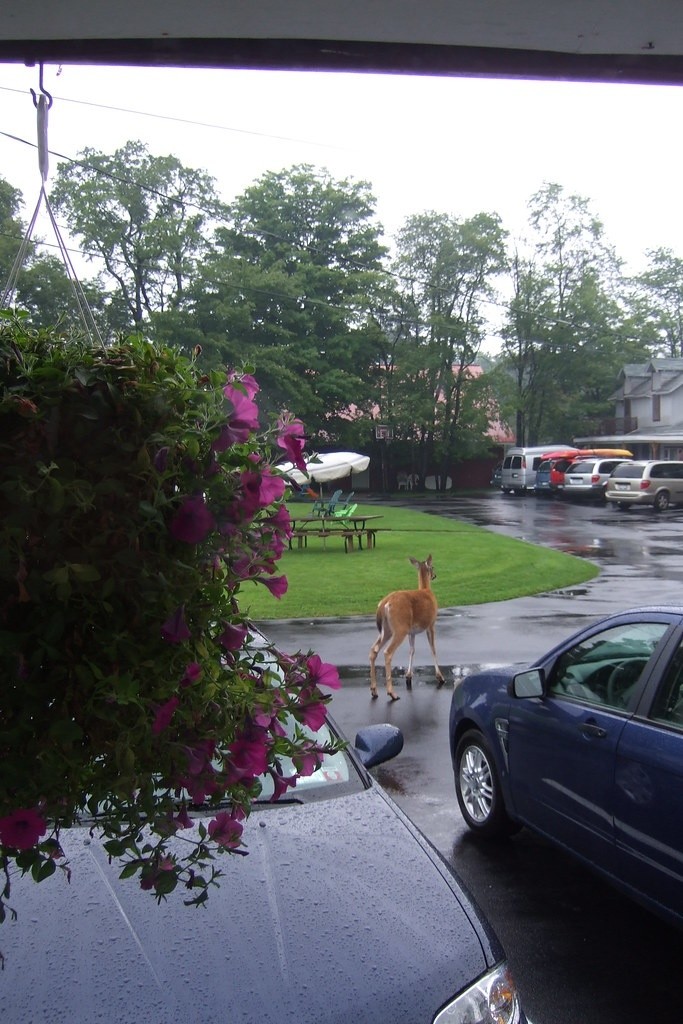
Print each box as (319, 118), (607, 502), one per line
(564, 459), (635, 505)
(605, 460), (683, 512)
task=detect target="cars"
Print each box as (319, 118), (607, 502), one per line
(0, 619), (536, 1024)
(448, 604), (683, 930)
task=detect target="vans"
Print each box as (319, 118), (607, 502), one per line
(501, 444), (579, 497)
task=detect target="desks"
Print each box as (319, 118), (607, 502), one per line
(252, 518), (299, 550)
(300, 516), (384, 551)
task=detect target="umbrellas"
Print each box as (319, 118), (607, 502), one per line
(276, 452), (370, 549)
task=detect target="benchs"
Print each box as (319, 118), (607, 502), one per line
(290, 528), (392, 553)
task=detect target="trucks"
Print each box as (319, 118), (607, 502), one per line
(533, 448), (633, 499)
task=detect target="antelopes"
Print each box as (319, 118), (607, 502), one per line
(369, 554), (446, 701)
